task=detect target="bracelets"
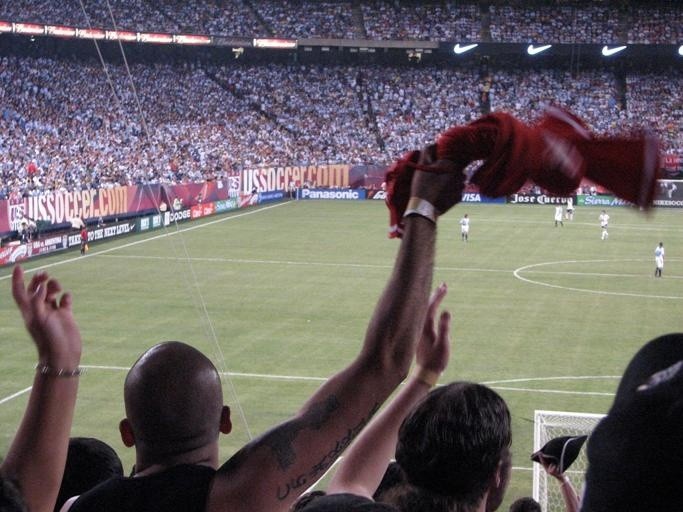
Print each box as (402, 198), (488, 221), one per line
(401, 197), (440, 226)
(36, 363), (80, 376)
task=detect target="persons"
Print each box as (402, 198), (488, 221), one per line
(598, 210), (609, 240)
(0, 265), (81, 512)
(554, 204), (563, 227)
(459, 214), (469, 241)
(565, 197), (575, 220)
(293, 284), (682, 511)
(19, 180), (309, 255)
(654, 242), (664, 278)
(0, 0), (682, 181)
(54, 437), (123, 510)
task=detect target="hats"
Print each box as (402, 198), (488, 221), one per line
(531, 433), (586, 474)
(586, 333), (681, 511)
